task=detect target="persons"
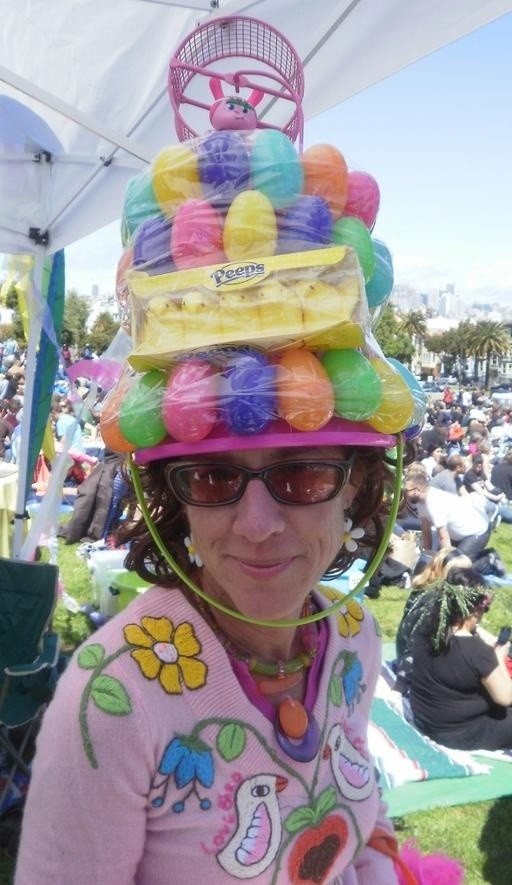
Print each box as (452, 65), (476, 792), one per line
(396, 544), (512, 751)
(362, 386), (511, 592)
(0, 336), (136, 546)
(12, 445), (414, 885)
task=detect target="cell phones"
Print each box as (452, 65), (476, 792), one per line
(497, 625), (512, 649)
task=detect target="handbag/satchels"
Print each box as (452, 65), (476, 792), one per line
(361, 554), (413, 588)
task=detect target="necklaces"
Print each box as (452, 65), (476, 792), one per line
(192, 570), (320, 762)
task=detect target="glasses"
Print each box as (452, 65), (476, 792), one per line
(162, 448), (357, 509)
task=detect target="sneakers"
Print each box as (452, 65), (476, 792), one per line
(486, 547), (505, 577)
